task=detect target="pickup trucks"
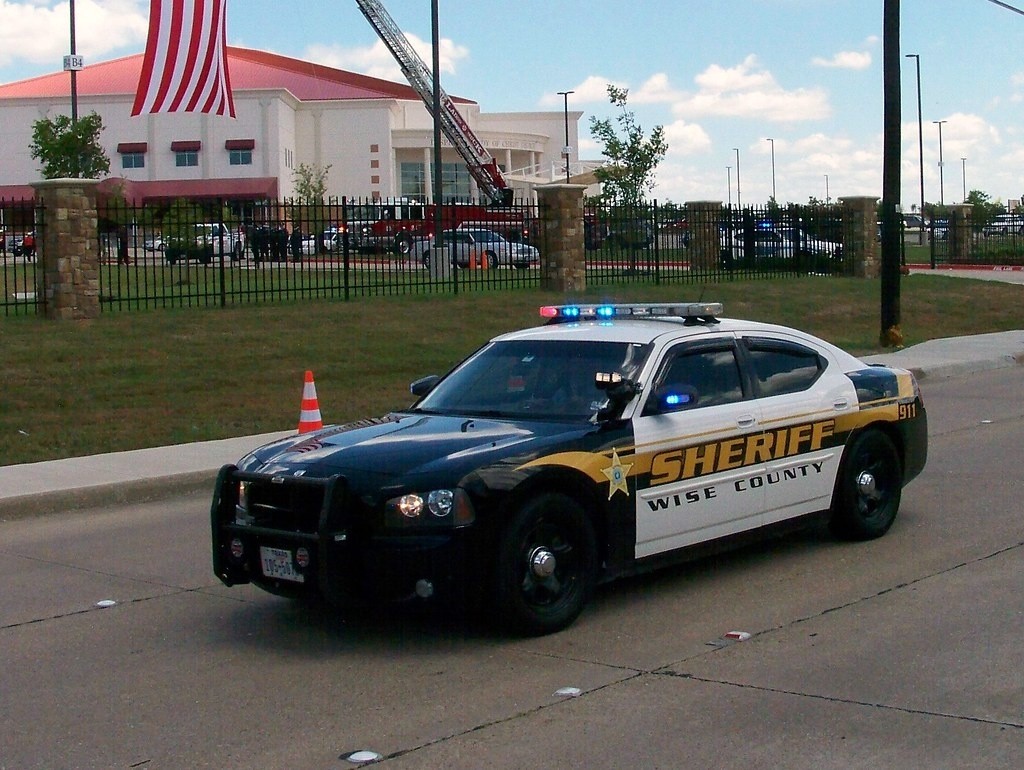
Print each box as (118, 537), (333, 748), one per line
(166, 222), (245, 263)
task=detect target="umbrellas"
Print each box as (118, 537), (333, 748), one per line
(98, 216), (121, 232)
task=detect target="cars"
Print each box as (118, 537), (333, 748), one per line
(612, 218), (654, 248)
(141, 235), (169, 250)
(410, 228), (540, 270)
(662, 218), (689, 233)
(290, 227), (344, 255)
(876, 215), (932, 243)
(8, 229), (37, 257)
(209, 304), (927, 638)
(719, 222), (844, 262)
(932, 221), (950, 240)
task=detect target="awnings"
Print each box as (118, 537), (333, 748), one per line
(172, 141), (201, 151)
(0, 177), (278, 209)
(225, 140), (254, 150)
(117, 143), (147, 153)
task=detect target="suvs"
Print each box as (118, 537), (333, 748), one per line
(983, 214), (1024, 240)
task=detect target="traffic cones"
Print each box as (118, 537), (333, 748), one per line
(481, 253), (487, 270)
(470, 251), (476, 270)
(299, 371), (324, 434)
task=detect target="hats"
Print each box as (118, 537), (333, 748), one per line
(272, 221), (277, 225)
(279, 222), (284, 227)
(293, 223), (299, 228)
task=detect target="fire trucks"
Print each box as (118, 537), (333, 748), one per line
(336, 0), (605, 253)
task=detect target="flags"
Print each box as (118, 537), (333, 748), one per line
(131, 0), (235, 118)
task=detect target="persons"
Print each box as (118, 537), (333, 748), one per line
(0, 230), (3, 252)
(117, 228), (128, 264)
(24, 233), (33, 263)
(553, 358), (605, 405)
(157, 231), (161, 239)
(583, 210), (589, 238)
(388, 225), (419, 251)
(237, 223), (301, 263)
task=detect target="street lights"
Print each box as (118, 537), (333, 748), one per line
(906, 54), (924, 235)
(726, 166), (733, 205)
(767, 138), (775, 204)
(558, 90), (575, 185)
(962, 157), (966, 202)
(733, 148), (740, 212)
(825, 175), (829, 206)
(934, 120), (947, 204)
